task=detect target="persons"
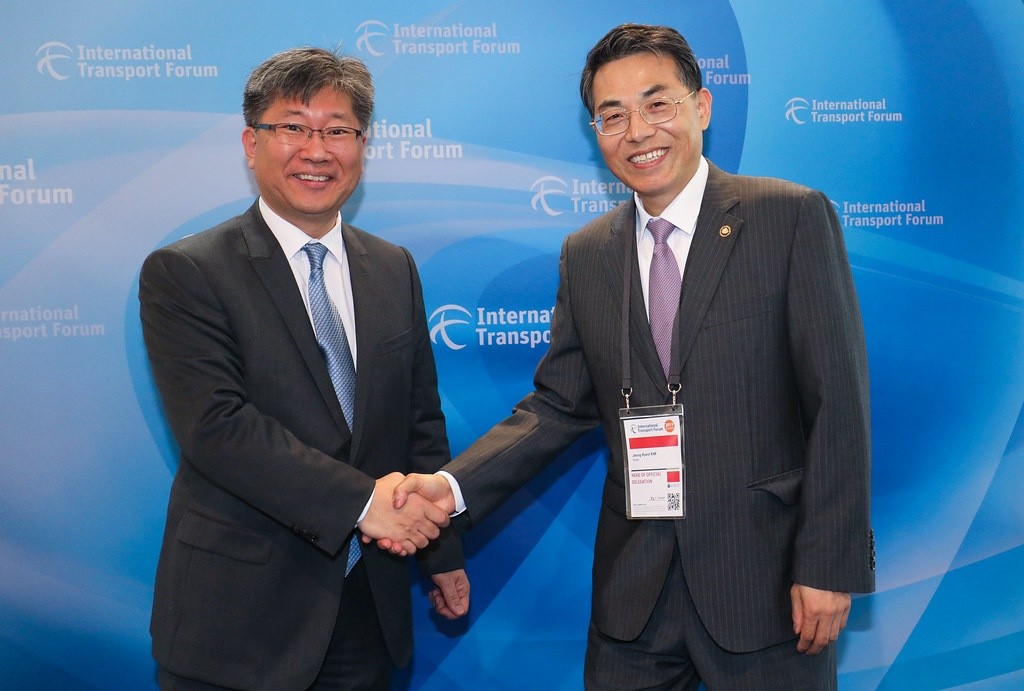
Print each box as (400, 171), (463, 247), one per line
(138, 48), (469, 691)
(363, 24), (875, 691)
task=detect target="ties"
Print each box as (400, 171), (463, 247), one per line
(646, 218), (682, 382)
(299, 242), (361, 576)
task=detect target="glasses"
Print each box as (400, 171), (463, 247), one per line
(253, 122), (363, 150)
(589, 91), (694, 136)
